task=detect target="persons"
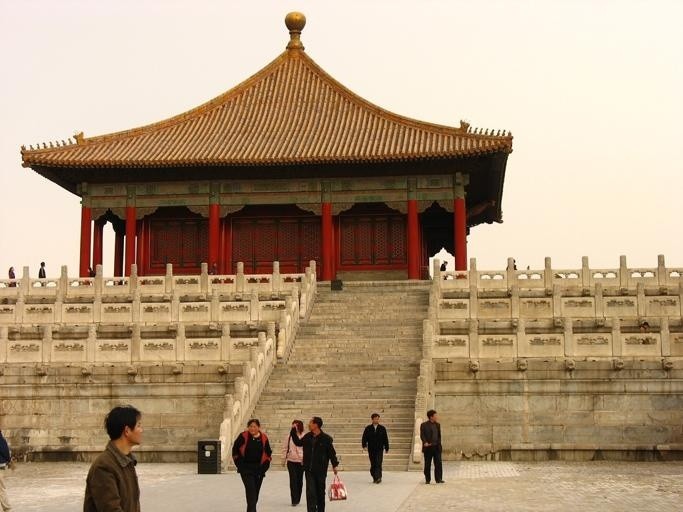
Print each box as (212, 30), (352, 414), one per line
(0, 429), (18, 512)
(37, 262), (47, 286)
(82, 403), (143, 512)
(7, 265), (16, 287)
(231, 418), (272, 512)
(360, 413), (389, 483)
(290, 416), (339, 512)
(280, 418), (306, 506)
(86, 265), (95, 285)
(209, 261), (217, 284)
(505, 259), (516, 270)
(438, 260), (449, 280)
(419, 408), (445, 484)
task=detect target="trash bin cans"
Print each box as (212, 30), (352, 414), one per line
(331, 280), (343, 290)
(198, 440), (221, 474)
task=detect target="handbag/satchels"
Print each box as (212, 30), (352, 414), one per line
(329, 474), (347, 501)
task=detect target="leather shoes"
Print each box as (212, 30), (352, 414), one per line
(425, 480), (430, 484)
(436, 479), (445, 483)
(376, 478), (381, 484)
